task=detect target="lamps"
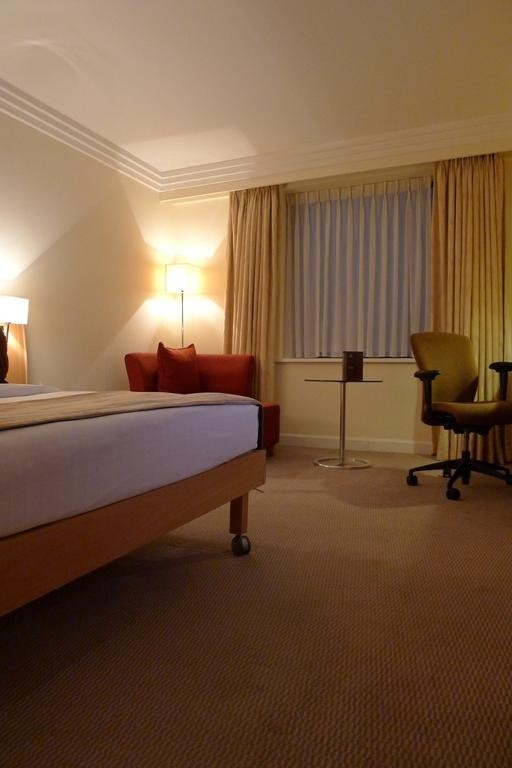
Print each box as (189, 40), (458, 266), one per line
(0, 297), (29, 341)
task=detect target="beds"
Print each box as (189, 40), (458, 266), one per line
(0, 383), (267, 617)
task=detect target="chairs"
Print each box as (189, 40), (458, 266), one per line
(406, 332), (511, 500)
(125, 353), (280, 456)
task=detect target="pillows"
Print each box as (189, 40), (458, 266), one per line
(156, 342), (201, 392)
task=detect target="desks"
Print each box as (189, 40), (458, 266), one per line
(304, 380), (383, 469)
